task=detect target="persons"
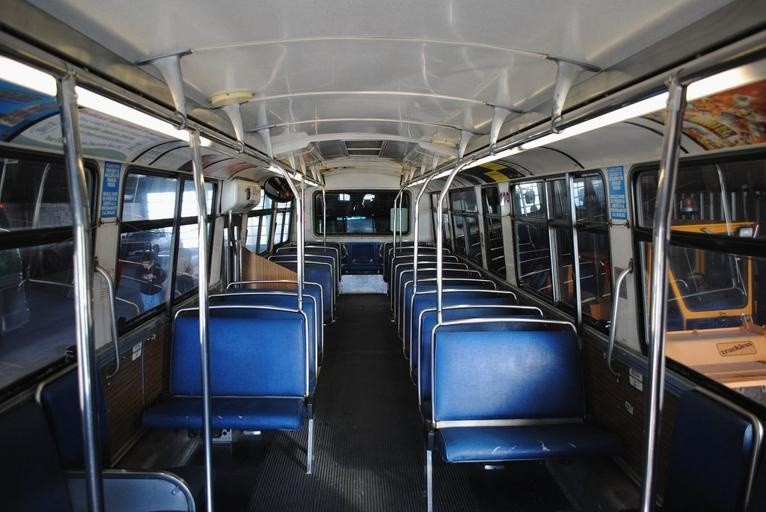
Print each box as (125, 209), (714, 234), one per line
(176, 241), (194, 293)
(133, 253), (167, 311)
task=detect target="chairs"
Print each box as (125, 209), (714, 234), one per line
(0, 363), (215, 511)
(656, 383), (764, 512)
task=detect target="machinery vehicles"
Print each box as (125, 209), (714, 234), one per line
(557, 220), (765, 403)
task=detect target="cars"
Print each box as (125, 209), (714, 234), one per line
(119, 229), (183, 261)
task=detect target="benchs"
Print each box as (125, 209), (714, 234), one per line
(381, 241), (519, 388)
(207, 280), (325, 409)
(416, 303), (545, 430)
(423, 317), (600, 512)
(141, 305), (316, 473)
(260, 241), (342, 325)
